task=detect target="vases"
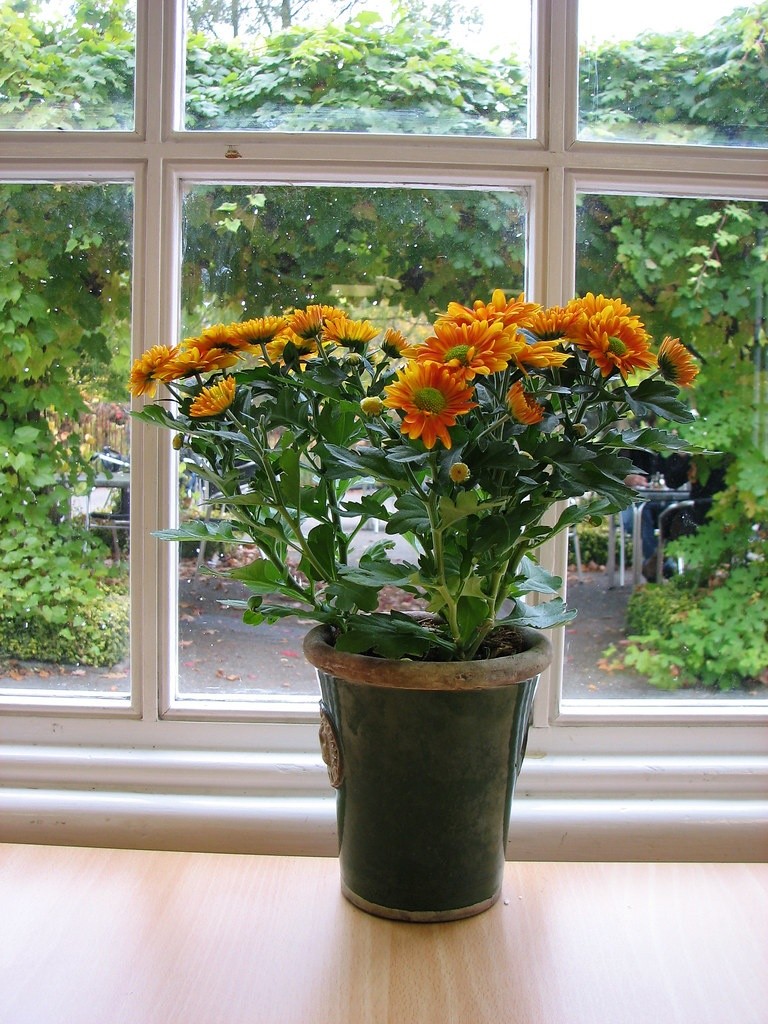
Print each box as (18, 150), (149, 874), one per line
(302, 613), (553, 926)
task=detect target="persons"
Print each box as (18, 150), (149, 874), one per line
(620, 448), (736, 583)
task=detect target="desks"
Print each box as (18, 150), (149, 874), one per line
(606, 489), (690, 590)
(54, 472), (129, 519)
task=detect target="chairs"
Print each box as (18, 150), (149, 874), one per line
(655, 498), (717, 585)
(337, 477), (379, 533)
(81, 452), (130, 569)
(195, 459), (269, 576)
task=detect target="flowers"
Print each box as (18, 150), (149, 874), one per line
(125, 288), (700, 659)
